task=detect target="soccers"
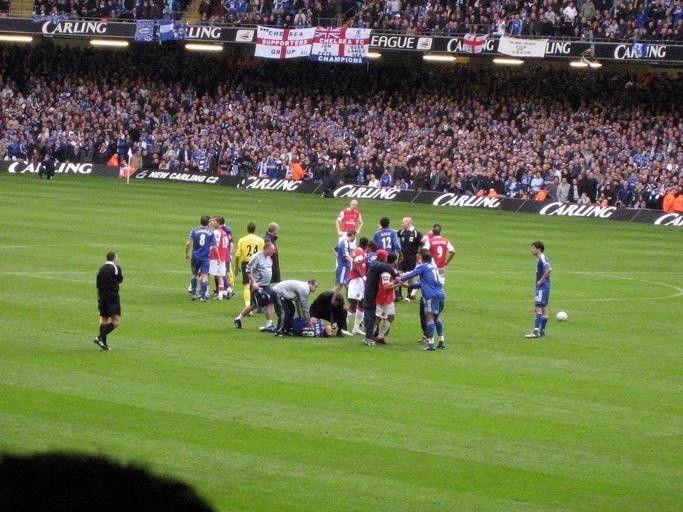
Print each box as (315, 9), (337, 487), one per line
(557, 311), (568, 322)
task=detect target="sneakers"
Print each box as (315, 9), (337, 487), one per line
(260, 323), (295, 338)
(188, 287), (236, 302)
(330, 321), (390, 348)
(524, 333), (545, 339)
(94, 336), (111, 351)
(394, 292), (417, 303)
(233, 318), (243, 330)
(417, 335), (446, 351)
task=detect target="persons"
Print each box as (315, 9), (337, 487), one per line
(182, 213), (455, 350)
(94, 252), (122, 351)
(523, 241), (551, 339)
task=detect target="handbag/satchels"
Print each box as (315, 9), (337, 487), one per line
(291, 316), (324, 338)
